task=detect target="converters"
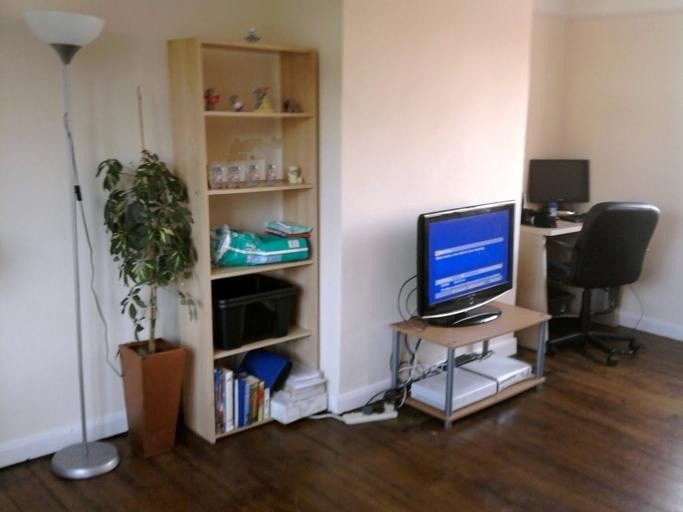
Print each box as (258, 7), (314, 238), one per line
(374, 400), (384, 413)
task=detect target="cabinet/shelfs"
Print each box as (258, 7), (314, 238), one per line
(168, 39), (322, 445)
(389, 299), (553, 429)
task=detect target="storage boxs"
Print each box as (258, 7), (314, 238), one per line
(212, 272), (297, 350)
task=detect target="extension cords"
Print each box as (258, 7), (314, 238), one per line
(342, 408), (398, 426)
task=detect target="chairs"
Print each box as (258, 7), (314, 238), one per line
(546, 201), (661, 367)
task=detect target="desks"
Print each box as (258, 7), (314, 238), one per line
(514, 222), (617, 353)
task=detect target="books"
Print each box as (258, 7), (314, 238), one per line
(269, 360), (327, 427)
(214, 365), (270, 432)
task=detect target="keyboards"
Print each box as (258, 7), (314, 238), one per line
(558, 213), (590, 223)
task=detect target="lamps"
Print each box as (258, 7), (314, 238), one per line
(22, 11), (120, 481)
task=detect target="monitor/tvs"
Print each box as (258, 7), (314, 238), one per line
(529, 159), (590, 217)
(416, 199), (515, 327)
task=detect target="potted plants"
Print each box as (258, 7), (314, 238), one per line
(96, 149), (202, 458)
